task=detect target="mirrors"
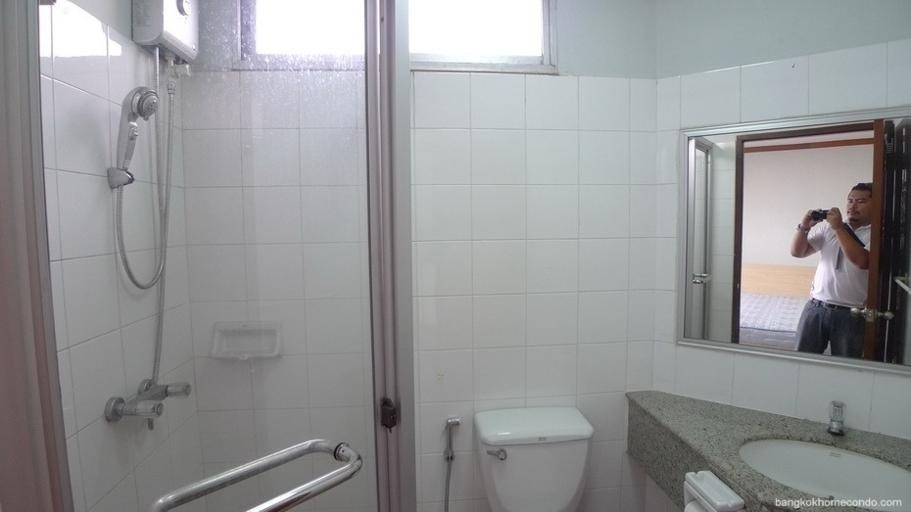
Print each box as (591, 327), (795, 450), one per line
(675, 105), (911, 375)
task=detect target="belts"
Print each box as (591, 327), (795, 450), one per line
(808, 296), (865, 312)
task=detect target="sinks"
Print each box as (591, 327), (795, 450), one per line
(737, 434), (906, 512)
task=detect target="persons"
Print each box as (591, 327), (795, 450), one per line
(792, 182), (874, 359)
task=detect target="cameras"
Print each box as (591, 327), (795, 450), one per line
(810, 209), (830, 221)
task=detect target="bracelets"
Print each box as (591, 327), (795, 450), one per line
(794, 223), (810, 235)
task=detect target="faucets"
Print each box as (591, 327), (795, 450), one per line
(826, 398), (846, 437)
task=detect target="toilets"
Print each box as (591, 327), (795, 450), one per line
(474, 405), (594, 512)
